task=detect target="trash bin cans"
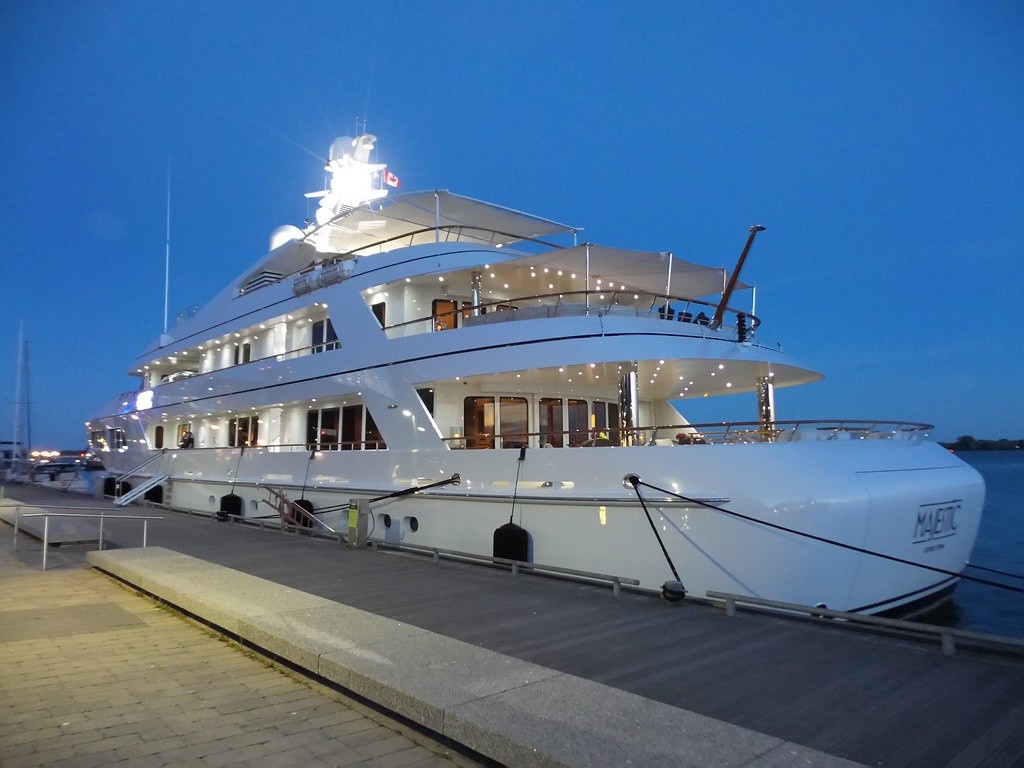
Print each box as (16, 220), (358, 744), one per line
(345, 497), (370, 549)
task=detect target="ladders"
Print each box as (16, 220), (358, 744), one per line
(112, 471), (171, 507)
(261, 485), (334, 540)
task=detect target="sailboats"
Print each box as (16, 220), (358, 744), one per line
(0, 319), (33, 485)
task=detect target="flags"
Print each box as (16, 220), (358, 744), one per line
(384, 169), (402, 188)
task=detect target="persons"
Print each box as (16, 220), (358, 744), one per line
(179, 431), (194, 449)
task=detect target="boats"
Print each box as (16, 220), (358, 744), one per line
(82, 131), (985, 621)
(27, 463), (89, 495)
